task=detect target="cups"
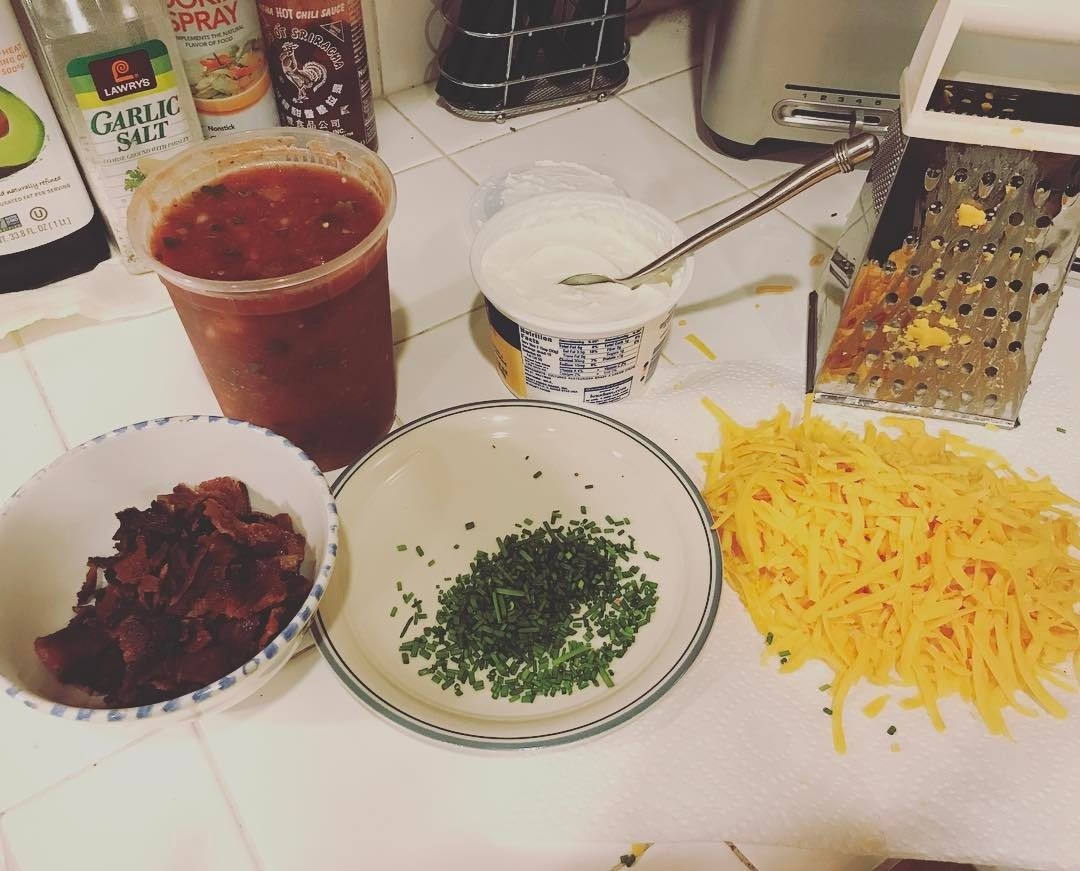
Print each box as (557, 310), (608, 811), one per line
(470, 190), (697, 407)
(125, 126), (398, 471)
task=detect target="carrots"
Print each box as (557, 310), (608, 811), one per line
(686, 334), (1079, 751)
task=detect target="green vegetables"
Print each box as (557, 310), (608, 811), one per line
(207, 38), (256, 72)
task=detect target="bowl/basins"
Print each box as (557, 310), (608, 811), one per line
(0, 417), (339, 730)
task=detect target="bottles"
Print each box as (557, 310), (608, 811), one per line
(1, 0), (112, 293)
(166, 0), (281, 139)
(256, 0), (379, 153)
(21, 0), (204, 274)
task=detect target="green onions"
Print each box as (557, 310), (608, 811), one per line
(389, 472), (660, 705)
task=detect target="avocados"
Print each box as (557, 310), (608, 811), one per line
(0, 87), (44, 180)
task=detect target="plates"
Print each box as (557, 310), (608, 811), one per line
(308, 399), (722, 751)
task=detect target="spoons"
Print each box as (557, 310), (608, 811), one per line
(557, 135), (881, 291)
